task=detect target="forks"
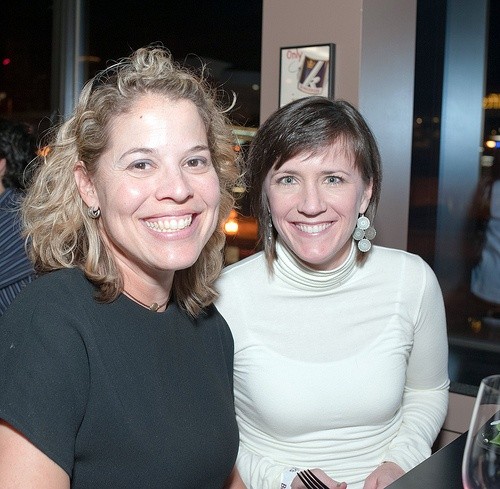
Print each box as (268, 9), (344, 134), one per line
(296, 469), (330, 489)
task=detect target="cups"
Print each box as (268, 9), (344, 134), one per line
(461, 375), (500, 489)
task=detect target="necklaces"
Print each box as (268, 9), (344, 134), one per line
(124, 290), (168, 311)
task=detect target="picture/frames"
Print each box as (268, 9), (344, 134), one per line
(278, 40), (335, 111)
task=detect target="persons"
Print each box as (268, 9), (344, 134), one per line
(469, 149), (500, 318)
(0, 120), (34, 317)
(0, 48), (248, 488)
(215, 96), (451, 489)
